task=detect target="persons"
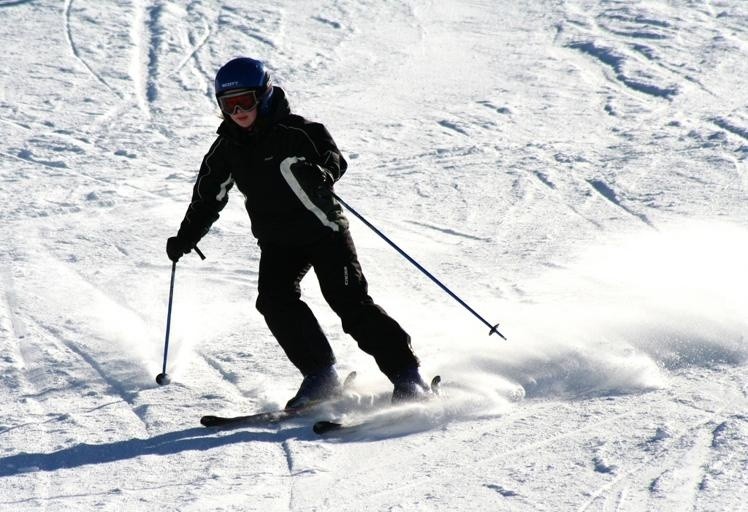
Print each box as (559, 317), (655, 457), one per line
(166, 57), (431, 413)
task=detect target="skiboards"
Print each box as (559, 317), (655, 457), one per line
(200, 392), (488, 434)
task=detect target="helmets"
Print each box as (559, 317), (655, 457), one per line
(215, 58), (273, 115)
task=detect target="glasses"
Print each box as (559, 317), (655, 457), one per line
(217, 74), (271, 115)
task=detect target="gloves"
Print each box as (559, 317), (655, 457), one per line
(167, 237), (190, 262)
(304, 162), (327, 189)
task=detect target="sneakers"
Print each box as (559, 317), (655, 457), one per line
(391, 369), (430, 403)
(284, 365), (351, 409)
